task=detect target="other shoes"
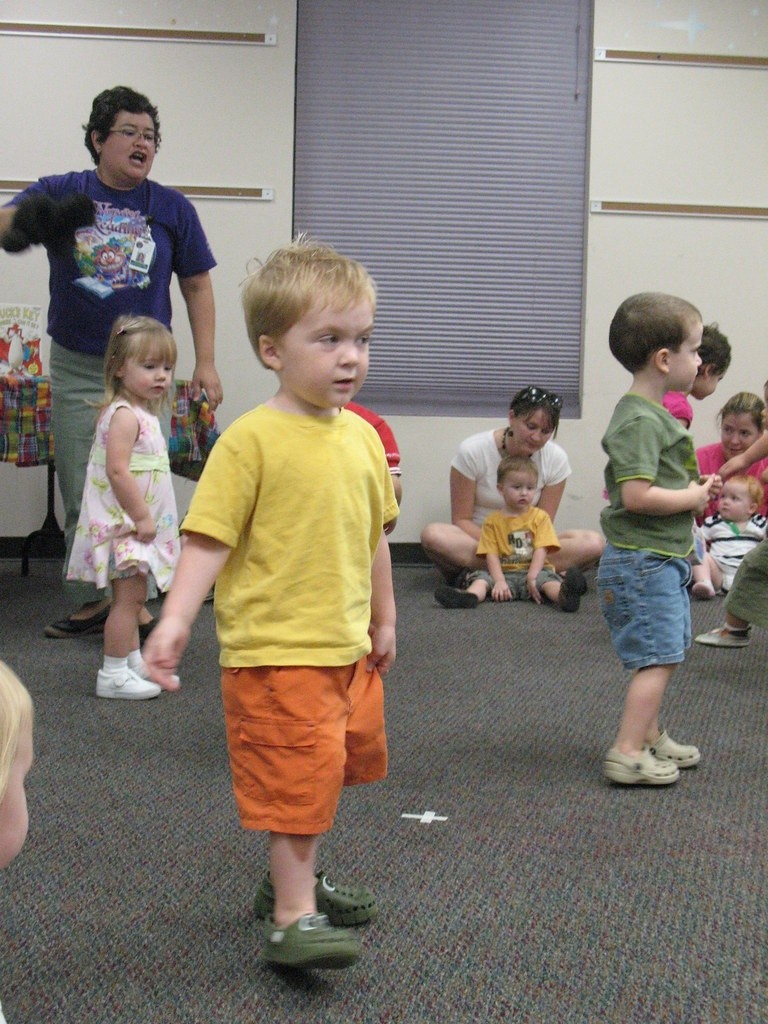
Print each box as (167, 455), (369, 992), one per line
(693, 582), (716, 599)
(43, 599), (111, 637)
(203, 585), (213, 602)
(435, 587), (479, 609)
(139, 615), (158, 641)
(556, 567), (587, 613)
(694, 619), (752, 647)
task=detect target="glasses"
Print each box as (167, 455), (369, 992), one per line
(105, 126), (161, 149)
(509, 386), (564, 418)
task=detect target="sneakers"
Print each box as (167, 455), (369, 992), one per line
(96, 665), (161, 700)
(133, 663), (180, 691)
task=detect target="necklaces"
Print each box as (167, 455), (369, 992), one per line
(502, 427), (533, 459)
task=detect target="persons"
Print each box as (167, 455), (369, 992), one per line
(598, 287), (725, 785)
(66, 314), (183, 700)
(690, 473), (767, 597)
(342, 398), (403, 537)
(694, 392), (768, 550)
(140, 228), (398, 968)
(693, 380), (768, 647)
(0, 661), (37, 872)
(0, 87), (225, 648)
(422, 384), (606, 588)
(602, 323), (732, 499)
(434, 455), (587, 612)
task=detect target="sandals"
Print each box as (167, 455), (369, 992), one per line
(258, 911), (362, 969)
(252, 868), (378, 920)
(647, 731), (700, 767)
(602, 744), (681, 785)
